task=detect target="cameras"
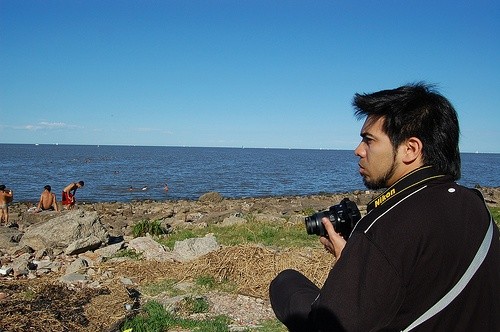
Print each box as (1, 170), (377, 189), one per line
(305, 198), (361, 241)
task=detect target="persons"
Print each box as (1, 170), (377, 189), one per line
(35, 184), (59, 212)
(141, 186), (147, 190)
(129, 187), (132, 190)
(0, 185), (13, 228)
(269, 84), (500, 332)
(163, 183), (170, 190)
(1, 185), (13, 222)
(62, 181), (85, 210)
